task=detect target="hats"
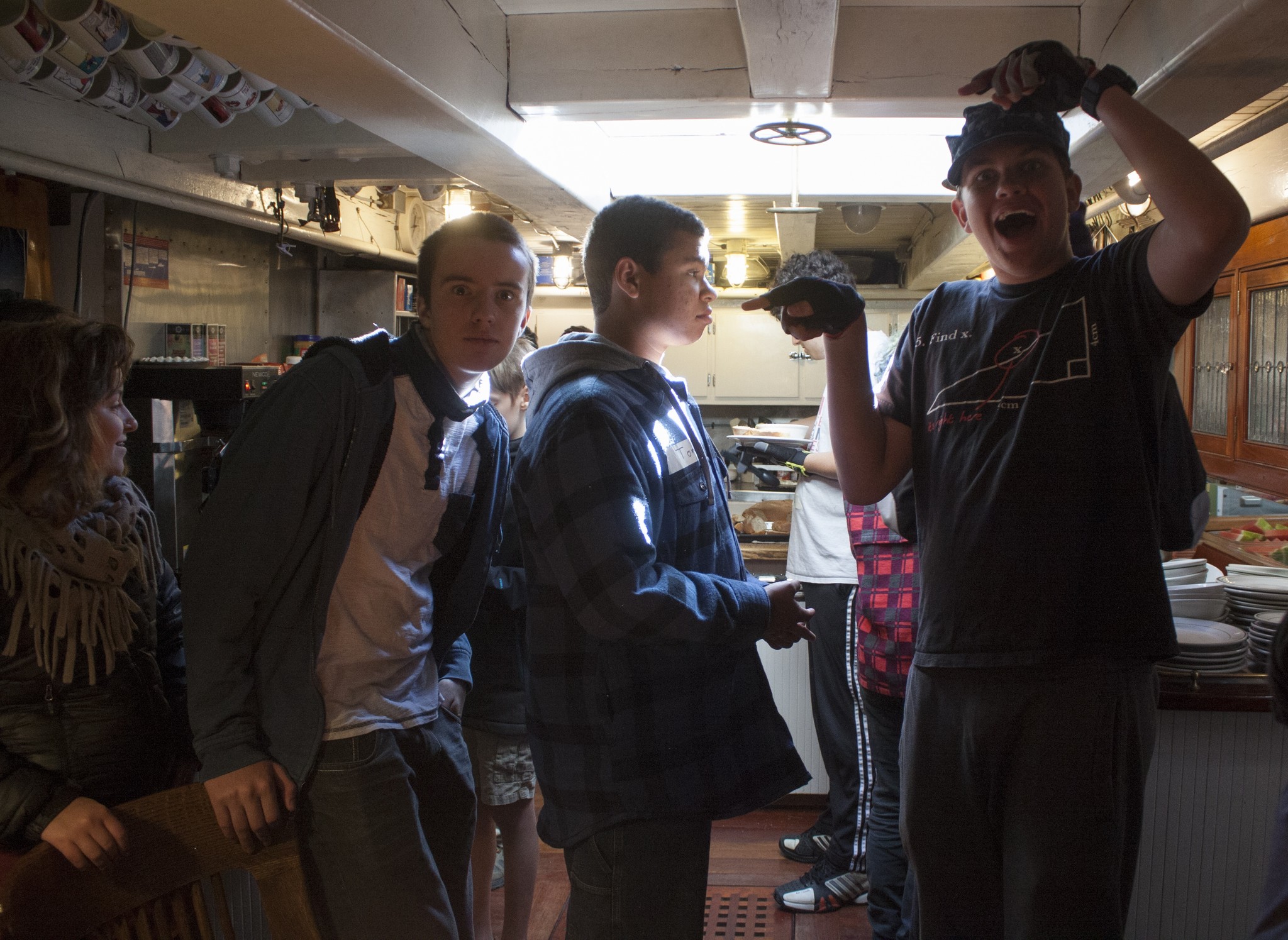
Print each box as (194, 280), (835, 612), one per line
(945, 93), (1071, 186)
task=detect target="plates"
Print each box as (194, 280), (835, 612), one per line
(1155, 555), (1288, 680)
(727, 435), (813, 451)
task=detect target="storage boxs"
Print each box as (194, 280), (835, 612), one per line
(292, 333), (322, 357)
(164, 319), (228, 368)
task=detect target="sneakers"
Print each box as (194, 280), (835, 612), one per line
(778, 810), (833, 863)
(774, 855), (872, 914)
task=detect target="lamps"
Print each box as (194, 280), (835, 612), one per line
(1117, 170), (1153, 225)
(712, 233), (761, 293)
(545, 234), (583, 295)
(837, 199), (886, 236)
(440, 185), (477, 224)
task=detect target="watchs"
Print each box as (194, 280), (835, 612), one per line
(1081, 63), (1138, 122)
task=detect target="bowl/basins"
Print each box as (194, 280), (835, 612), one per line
(755, 423), (809, 441)
(722, 470), (737, 482)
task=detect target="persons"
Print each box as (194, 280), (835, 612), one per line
(181, 210), (535, 940)
(842, 338), (920, 940)
(512, 197), (817, 940)
(754, 250), (895, 911)
(463, 338), (540, 940)
(0, 289), (181, 871)
(741, 39), (1251, 940)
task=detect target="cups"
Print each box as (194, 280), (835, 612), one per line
(1, 1), (345, 130)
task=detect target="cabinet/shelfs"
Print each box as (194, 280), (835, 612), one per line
(540, 296), (711, 407)
(713, 307), (896, 410)
(1167, 207), (1288, 506)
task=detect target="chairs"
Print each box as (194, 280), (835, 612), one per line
(4, 779), (328, 940)
